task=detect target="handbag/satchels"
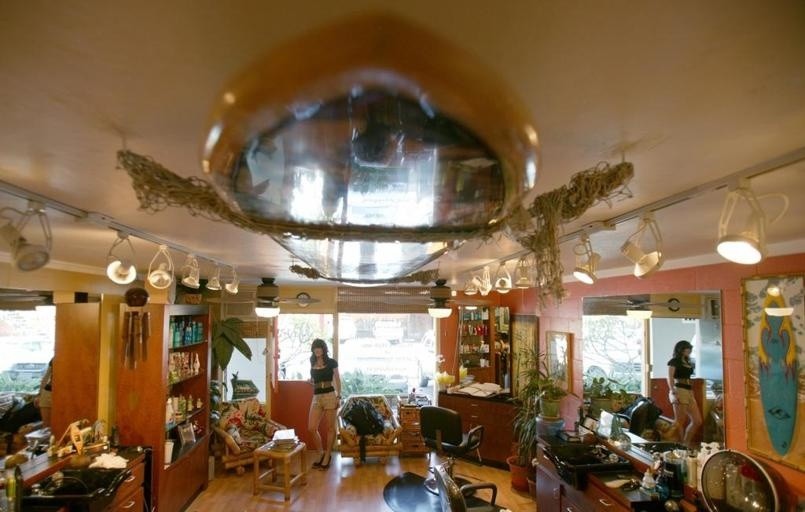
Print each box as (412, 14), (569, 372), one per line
(358, 400), (383, 433)
(620, 397), (654, 434)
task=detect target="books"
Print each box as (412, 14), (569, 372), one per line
(458, 387), (494, 398)
(268, 428), (300, 453)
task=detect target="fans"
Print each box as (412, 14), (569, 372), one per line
(204, 276), (321, 305)
(582, 294), (707, 310)
(379, 279), (490, 307)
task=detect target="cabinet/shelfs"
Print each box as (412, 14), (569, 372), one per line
(118, 303), (212, 512)
(438, 301), (530, 470)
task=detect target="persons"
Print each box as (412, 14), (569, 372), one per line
(34, 350), (54, 427)
(306, 338), (341, 471)
(666, 340), (702, 446)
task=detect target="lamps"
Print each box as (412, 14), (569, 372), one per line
(426, 298), (453, 320)
(626, 293), (654, 320)
(0, 180), (240, 296)
(253, 303), (280, 319)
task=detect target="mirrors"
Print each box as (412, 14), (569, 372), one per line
(0, 289), (118, 482)
(580, 289), (726, 452)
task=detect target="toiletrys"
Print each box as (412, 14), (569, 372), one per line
(459, 324), (487, 336)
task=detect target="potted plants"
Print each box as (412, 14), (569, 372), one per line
(507, 328), (569, 498)
(583, 374), (636, 417)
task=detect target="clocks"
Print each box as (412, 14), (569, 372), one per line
(296, 292), (312, 308)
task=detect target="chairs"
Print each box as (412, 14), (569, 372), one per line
(400, 405), (433, 460)
(420, 405), (486, 497)
(336, 394), (403, 468)
(431, 464), (508, 511)
(209, 399), (287, 477)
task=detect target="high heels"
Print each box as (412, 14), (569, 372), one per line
(312, 454), (325, 469)
(320, 456), (331, 471)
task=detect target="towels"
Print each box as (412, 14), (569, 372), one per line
(122, 308), (151, 372)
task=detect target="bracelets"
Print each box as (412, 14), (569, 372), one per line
(336, 396), (341, 400)
(669, 390), (678, 395)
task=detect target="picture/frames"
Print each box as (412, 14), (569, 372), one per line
(739, 272), (805, 472)
(544, 330), (574, 397)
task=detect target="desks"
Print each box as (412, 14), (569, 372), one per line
(1, 439), (152, 512)
(253, 438), (307, 500)
(532, 443), (720, 512)
